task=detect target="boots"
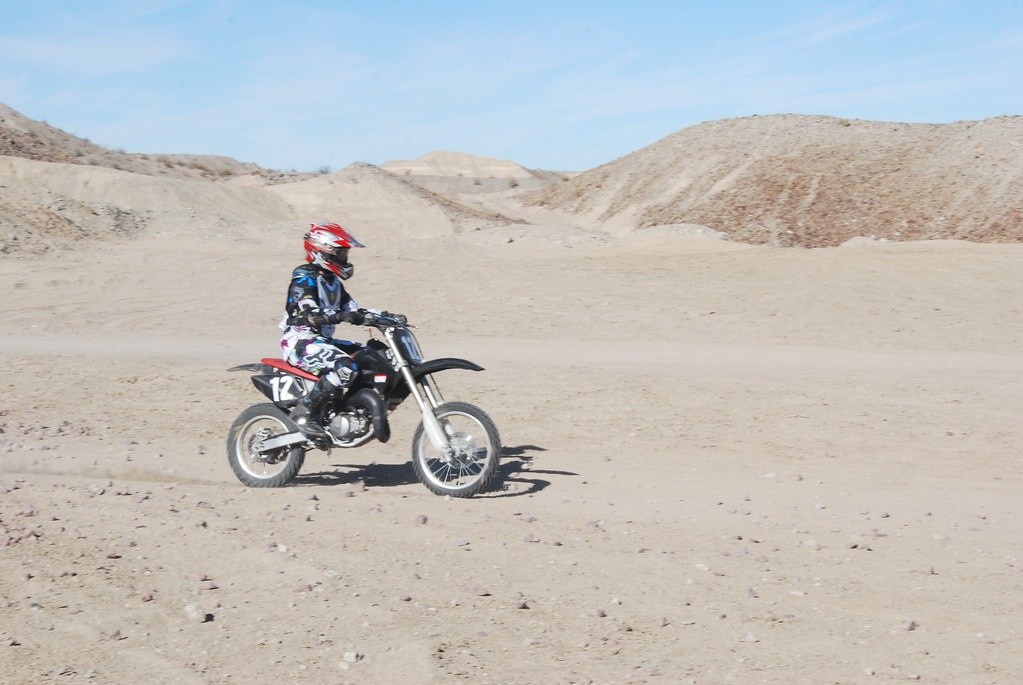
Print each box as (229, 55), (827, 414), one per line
(288, 375), (341, 439)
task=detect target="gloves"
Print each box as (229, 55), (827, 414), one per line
(381, 311), (407, 324)
(340, 311), (364, 325)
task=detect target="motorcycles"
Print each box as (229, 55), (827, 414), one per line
(225, 306), (501, 498)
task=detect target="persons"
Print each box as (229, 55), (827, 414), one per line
(279, 222), (407, 445)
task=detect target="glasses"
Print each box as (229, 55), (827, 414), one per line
(331, 247), (349, 260)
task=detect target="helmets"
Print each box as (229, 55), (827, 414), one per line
(303, 223), (365, 281)
